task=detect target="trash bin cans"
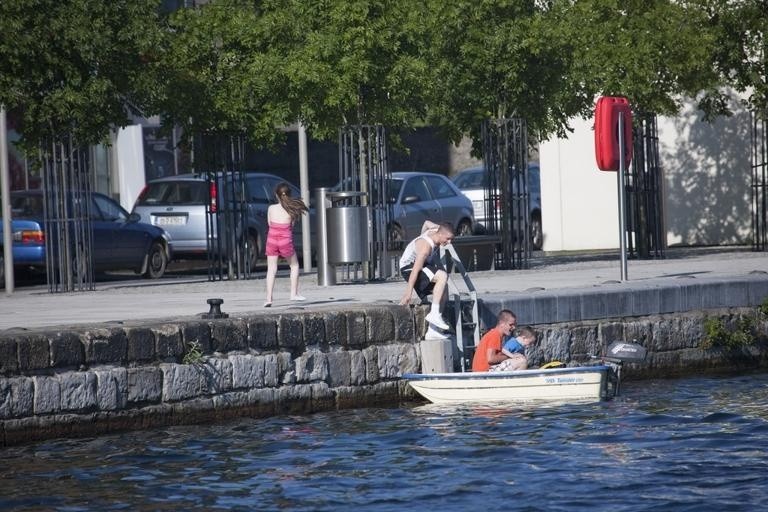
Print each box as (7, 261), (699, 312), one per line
(314, 185), (368, 287)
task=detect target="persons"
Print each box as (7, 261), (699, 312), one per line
(471, 309), (528, 371)
(262, 181), (313, 307)
(398, 218), (457, 342)
(502, 326), (537, 360)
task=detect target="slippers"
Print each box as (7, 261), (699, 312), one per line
(264, 302), (272, 307)
(290, 295), (306, 300)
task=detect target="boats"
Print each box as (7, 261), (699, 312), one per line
(402, 337), (649, 405)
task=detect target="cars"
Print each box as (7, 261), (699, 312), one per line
(129, 171), (321, 272)
(440, 162), (544, 250)
(1, 183), (172, 285)
(319, 172), (476, 251)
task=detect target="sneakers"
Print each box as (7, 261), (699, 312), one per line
(425, 330), (452, 340)
(424, 312), (449, 330)
